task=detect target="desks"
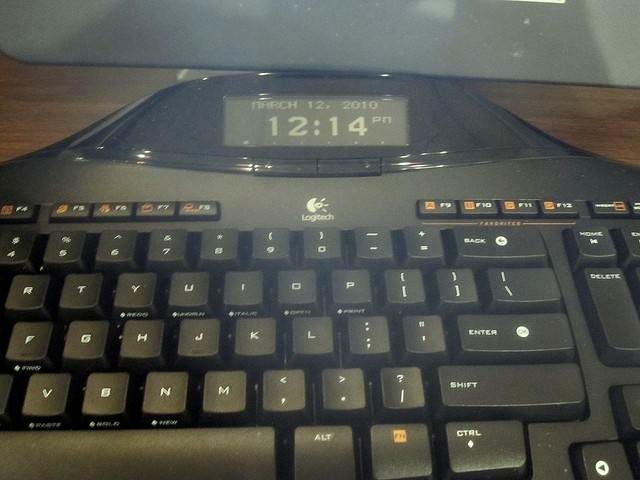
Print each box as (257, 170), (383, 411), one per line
(2, 62), (639, 164)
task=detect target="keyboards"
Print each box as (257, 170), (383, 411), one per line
(0, 72), (640, 479)
